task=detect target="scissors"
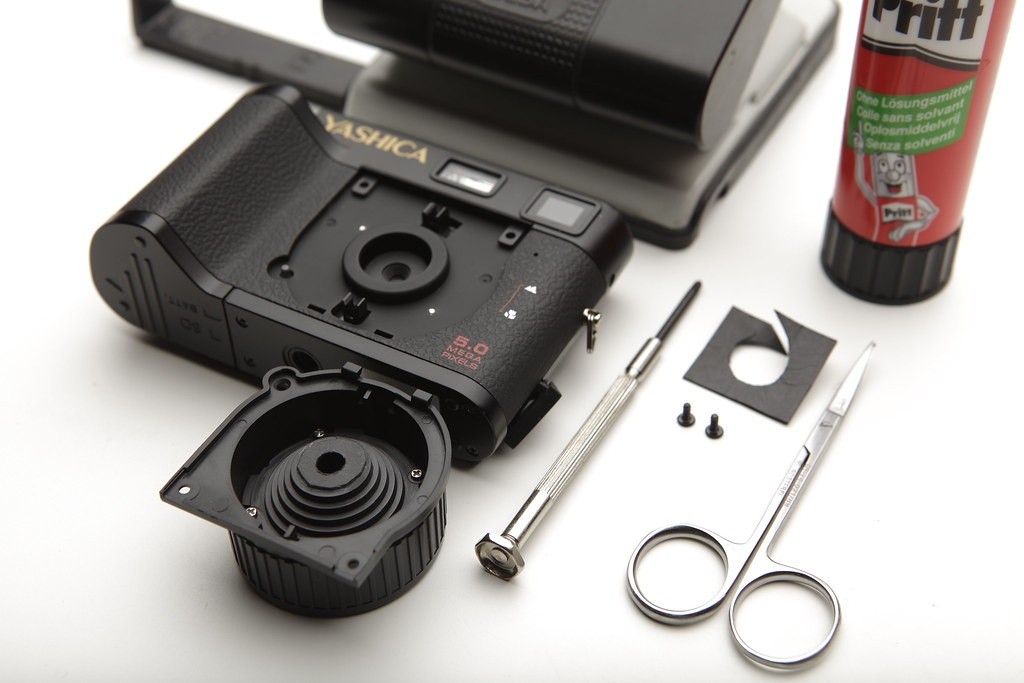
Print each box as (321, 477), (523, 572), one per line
(622, 335), (883, 674)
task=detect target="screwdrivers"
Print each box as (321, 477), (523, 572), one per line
(474, 274), (702, 580)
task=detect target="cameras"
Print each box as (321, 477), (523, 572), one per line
(89, 83), (634, 472)
